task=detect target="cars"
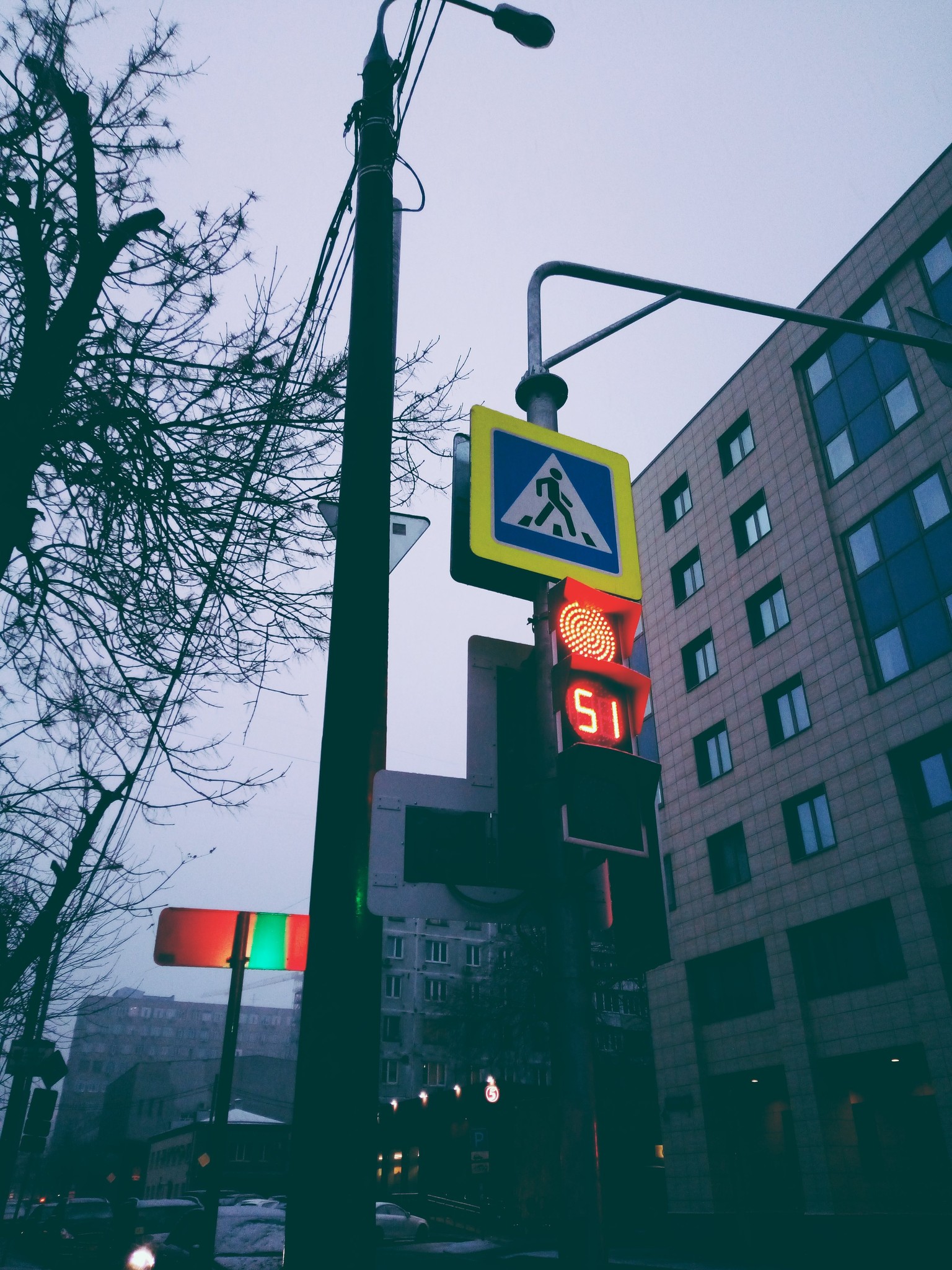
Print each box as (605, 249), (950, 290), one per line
(372, 1202), (430, 1240)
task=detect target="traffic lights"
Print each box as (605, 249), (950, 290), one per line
(534, 576), (665, 860)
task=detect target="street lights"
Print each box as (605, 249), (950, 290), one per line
(285, 1), (555, 1270)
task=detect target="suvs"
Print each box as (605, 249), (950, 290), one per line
(110, 1189), (287, 1253)
(28, 1196), (118, 1241)
